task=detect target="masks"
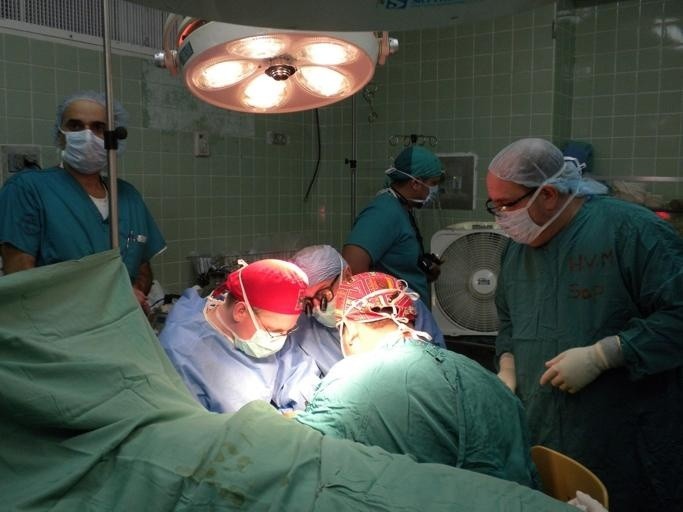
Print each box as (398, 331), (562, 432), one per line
(493, 164), (583, 246)
(340, 280), (434, 362)
(214, 257), (288, 359)
(381, 166), (442, 211)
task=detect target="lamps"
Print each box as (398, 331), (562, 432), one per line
(154, 13), (399, 114)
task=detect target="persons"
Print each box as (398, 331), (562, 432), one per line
(294, 272), (538, 490)
(484, 137), (682, 508)
(0, 88), (166, 315)
(342, 146), (446, 314)
(288, 244), (446, 353)
(159, 259), (320, 417)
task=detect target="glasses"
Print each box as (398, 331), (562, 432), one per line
(485, 187), (546, 218)
(297, 272), (344, 313)
(245, 302), (299, 340)
(55, 126), (108, 177)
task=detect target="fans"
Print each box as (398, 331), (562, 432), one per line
(429, 220), (510, 338)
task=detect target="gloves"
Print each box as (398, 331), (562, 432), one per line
(539, 335), (622, 395)
(496, 352), (518, 398)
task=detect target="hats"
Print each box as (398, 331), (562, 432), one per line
(56, 90), (131, 129)
(307, 296), (341, 330)
(387, 145), (445, 183)
(288, 242), (349, 287)
(211, 257), (310, 317)
(332, 270), (421, 325)
(486, 137), (610, 198)
